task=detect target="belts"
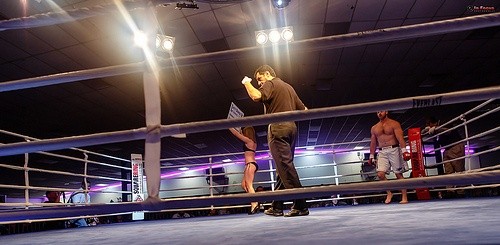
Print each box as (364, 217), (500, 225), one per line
(378, 145), (398, 150)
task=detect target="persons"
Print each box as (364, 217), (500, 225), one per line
(420, 119), (465, 200)
(241, 64), (309, 217)
(229, 126), (260, 215)
(368, 112), (412, 204)
(44, 182), (500, 228)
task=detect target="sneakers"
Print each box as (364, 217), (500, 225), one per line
(284, 208), (309, 217)
(264, 208), (284, 216)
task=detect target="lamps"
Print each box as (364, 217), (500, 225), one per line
(156, 34), (176, 52)
(254, 27), (294, 45)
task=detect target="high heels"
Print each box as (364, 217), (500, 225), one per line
(248, 206), (260, 215)
